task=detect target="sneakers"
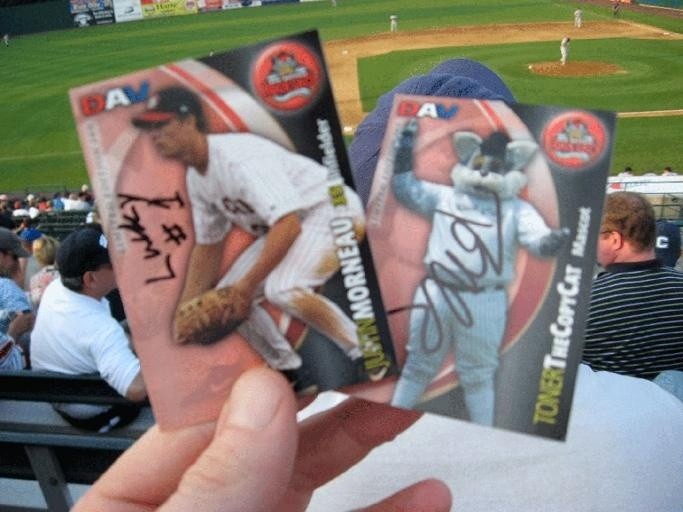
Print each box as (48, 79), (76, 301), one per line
(358, 349), (389, 383)
(283, 366), (319, 395)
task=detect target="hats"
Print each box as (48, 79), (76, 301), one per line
(56, 228), (111, 277)
(131, 86), (201, 131)
(0, 227), (41, 257)
(652, 218), (683, 269)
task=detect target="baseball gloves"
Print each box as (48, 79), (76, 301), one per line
(174, 286), (252, 346)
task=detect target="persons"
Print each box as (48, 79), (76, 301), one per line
(574, 7), (584, 28)
(129, 81), (573, 430)
(3, 32), (8, 47)
(583, 192), (683, 378)
(655, 222), (682, 269)
(390, 13), (399, 32)
(560, 37), (570, 64)
(1, 188), (149, 434)
(613, 3), (620, 18)
(617, 167), (678, 178)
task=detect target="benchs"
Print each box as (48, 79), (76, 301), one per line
(2, 209), (158, 512)
